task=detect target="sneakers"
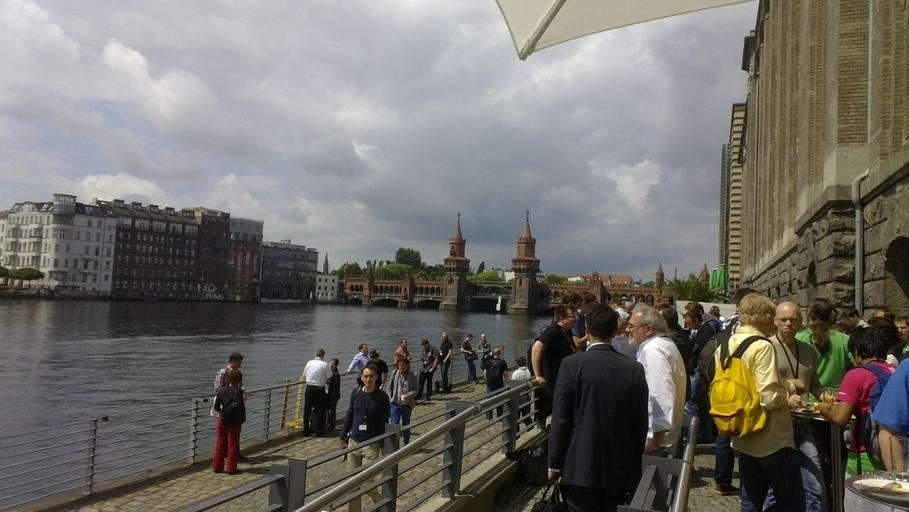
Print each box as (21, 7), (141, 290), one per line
(714, 484), (742, 496)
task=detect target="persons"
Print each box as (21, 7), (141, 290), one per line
(460, 333), (491, 384)
(213, 352), (249, 463)
(418, 332), (453, 401)
(531, 289), (909, 512)
(212, 370), (246, 473)
(302, 338), (419, 512)
(484, 348), (533, 441)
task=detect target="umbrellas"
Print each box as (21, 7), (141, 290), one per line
(498, 0), (768, 60)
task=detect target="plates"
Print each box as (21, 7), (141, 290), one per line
(854, 476), (909, 502)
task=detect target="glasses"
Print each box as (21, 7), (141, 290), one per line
(625, 322), (644, 330)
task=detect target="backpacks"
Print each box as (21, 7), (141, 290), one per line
(697, 316), (740, 387)
(219, 397), (246, 428)
(708, 333), (777, 439)
(856, 363), (897, 471)
(487, 358), (504, 388)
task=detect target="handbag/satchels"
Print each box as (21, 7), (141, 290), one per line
(530, 473), (571, 512)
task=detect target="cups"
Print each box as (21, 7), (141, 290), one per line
(888, 435), (908, 481)
(823, 386), (836, 404)
